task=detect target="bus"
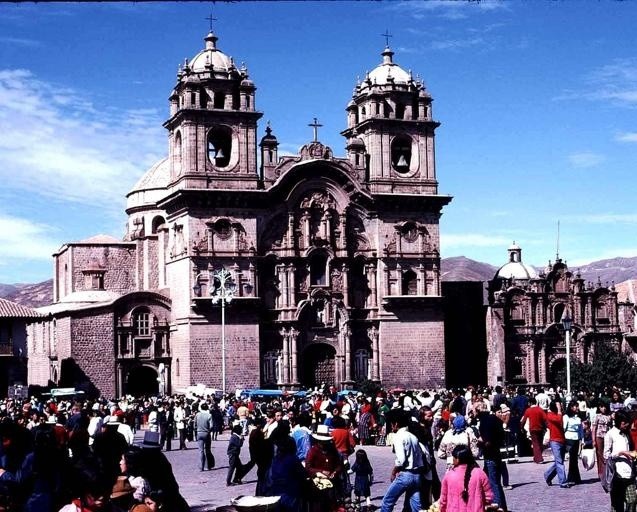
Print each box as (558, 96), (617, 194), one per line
(235, 385), (360, 404)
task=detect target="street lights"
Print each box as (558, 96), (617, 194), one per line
(191, 265), (254, 403)
(560, 308), (576, 394)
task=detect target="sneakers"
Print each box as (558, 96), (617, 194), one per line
(503, 486), (513, 491)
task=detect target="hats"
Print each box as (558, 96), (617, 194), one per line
(110, 476), (138, 500)
(311, 423), (334, 442)
(105, 416), (122, 426)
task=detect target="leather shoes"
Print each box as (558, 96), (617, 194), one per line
(545, 478), (571, 488)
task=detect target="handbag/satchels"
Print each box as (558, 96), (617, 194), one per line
(578, 443), (597, 471)
